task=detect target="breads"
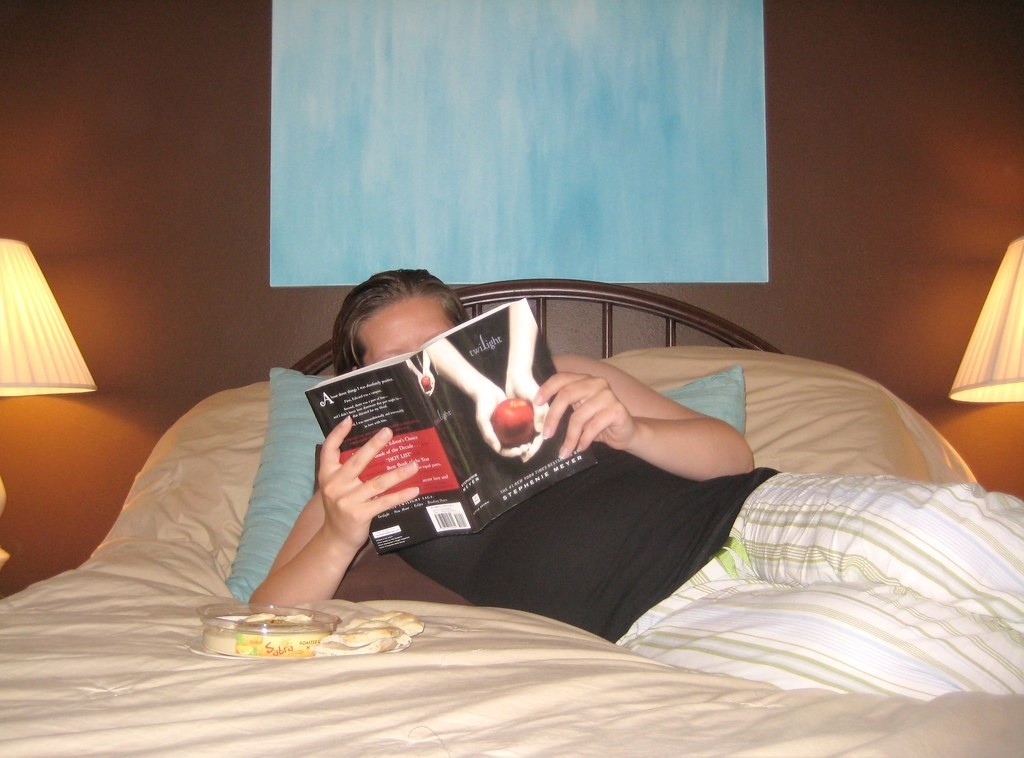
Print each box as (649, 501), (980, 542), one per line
(234, 611), (329, 658)
(312, 611), (425, 657)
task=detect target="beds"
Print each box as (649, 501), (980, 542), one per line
(0, 280), (1024, 757)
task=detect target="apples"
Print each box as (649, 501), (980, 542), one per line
(490, 397), (534, 448)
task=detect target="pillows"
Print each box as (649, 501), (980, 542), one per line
(226, 366), (747, 600)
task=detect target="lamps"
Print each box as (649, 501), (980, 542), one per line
(948, 236), (1024, 405)
(0, 239), (97, 568)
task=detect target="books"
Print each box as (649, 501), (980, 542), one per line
(303, 296), (600, 555)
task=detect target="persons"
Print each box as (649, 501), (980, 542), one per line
(249, 270), (1024, 701)
